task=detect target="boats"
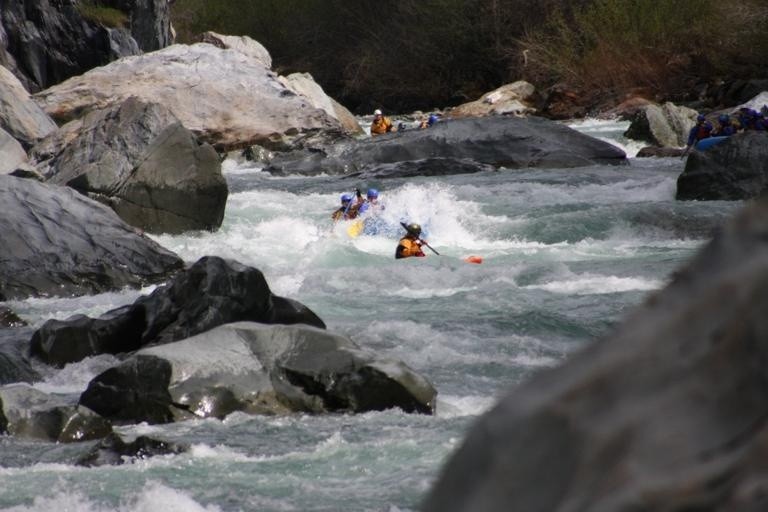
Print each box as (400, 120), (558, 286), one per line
(332, 203), (429, 242)
(696, 136), (726, 151)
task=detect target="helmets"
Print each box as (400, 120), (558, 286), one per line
(374, 109), (439, 129)
(696, 106), (758, 122)
(342, 189), (378, 203)
(408, 223), (422, 233)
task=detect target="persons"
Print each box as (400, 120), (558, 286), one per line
(395, 223), (427, 258)
(368, 108), (447, 137)
(331, 186), (363, 221)
(679, 103), (767, 161)
(358, 185), (385, 215)
